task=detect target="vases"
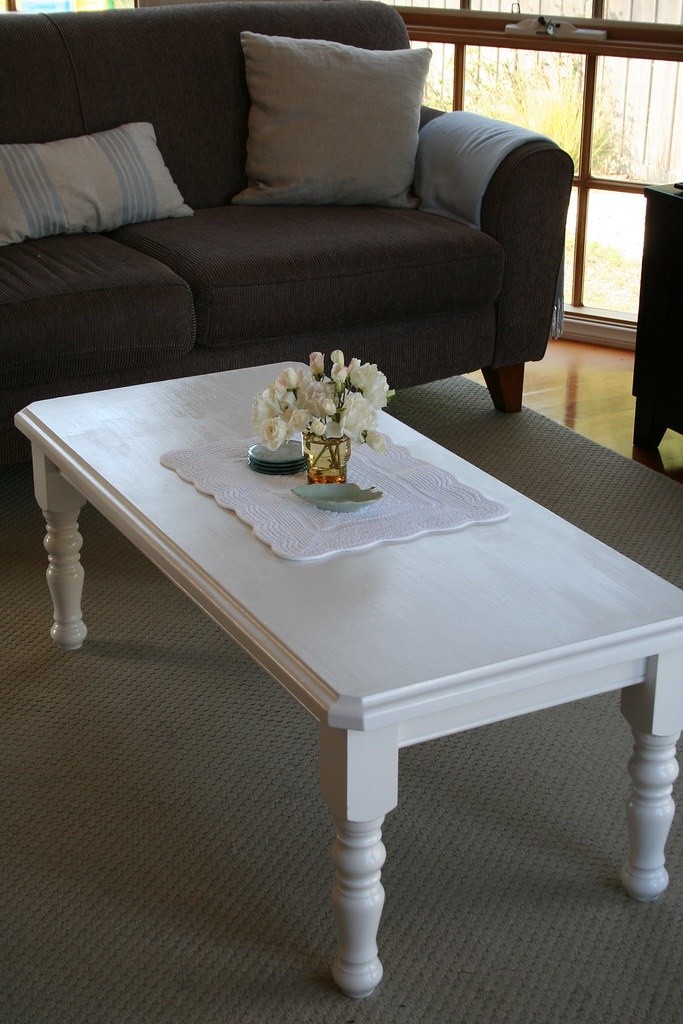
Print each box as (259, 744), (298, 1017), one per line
(302, 432), (351, 485)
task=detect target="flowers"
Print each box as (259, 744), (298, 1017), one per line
(250, 349), (397, 457)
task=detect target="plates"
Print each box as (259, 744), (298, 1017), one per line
(291, 483), (385, 513)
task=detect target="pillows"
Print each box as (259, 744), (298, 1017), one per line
(230, 31), (432, 209)
(0, 121), (195, 247)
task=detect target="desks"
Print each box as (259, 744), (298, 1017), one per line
(632, 182), (683, 452)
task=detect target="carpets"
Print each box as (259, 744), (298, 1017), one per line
(0, 378), (683, 1024)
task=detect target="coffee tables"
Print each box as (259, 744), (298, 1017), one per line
(13, 359), (683, 1002)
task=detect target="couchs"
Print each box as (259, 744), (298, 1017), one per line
(0, 0), (575, 467)
